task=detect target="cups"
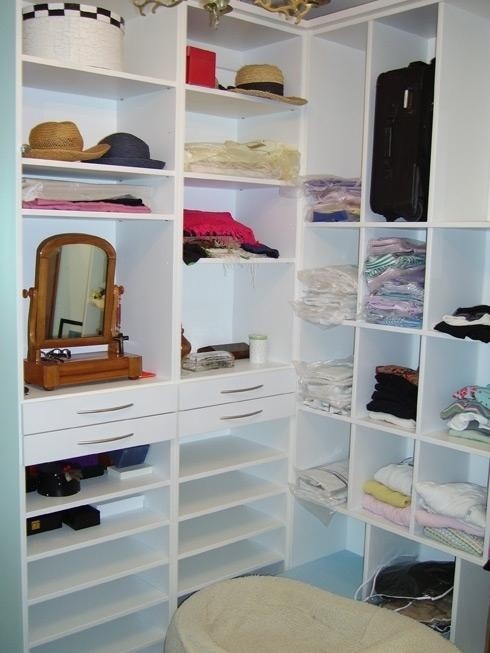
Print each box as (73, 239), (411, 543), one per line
(249, 335), (267, 364)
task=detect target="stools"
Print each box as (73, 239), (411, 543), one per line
(166, 573), (465, 651)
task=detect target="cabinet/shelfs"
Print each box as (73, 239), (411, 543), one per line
(301, 4), (490, 614)
(2, 2), (178, 647)
(178, 0), (304, 606)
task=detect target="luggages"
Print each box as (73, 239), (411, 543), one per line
(370, 57), (436, 222)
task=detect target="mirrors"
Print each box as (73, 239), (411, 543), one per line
(19, 227), (144, 387)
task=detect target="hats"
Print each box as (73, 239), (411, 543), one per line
(81, 133), (166, 168)
(215, 64), (308, 106)
(21, 122), (111, 162)
